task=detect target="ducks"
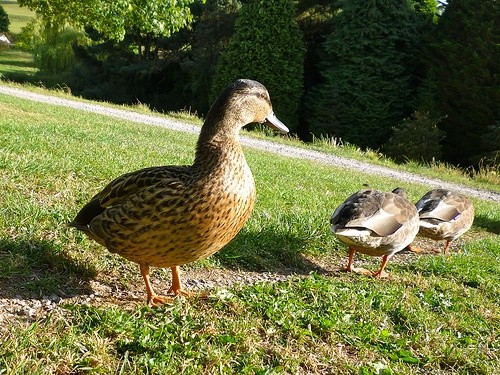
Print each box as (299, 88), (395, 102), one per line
(70, 78), (290, 307)
(415, 188), (476, 255)
(330, 187), (421, 278)
